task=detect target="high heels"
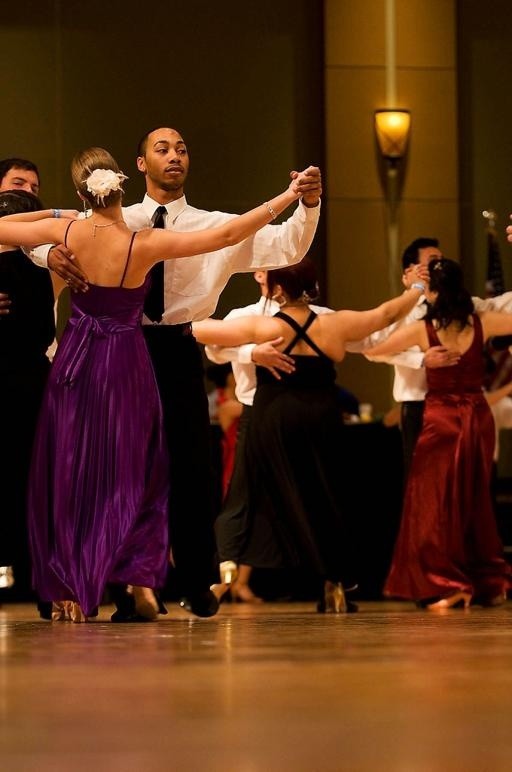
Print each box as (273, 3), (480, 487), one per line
(49, 601), (85, 622)
(318, 583), (358, 614)
(428, 593), (471, 610)
(230, 585), (263, 605)
(484, 589), (507, 604)
(111, 585), (159, 620)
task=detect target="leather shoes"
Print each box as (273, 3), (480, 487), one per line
(174, 578), (219, 616)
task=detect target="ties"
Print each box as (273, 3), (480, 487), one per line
(143, 208), (167, 324)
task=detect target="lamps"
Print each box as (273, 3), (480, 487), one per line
(372, 107), (415, 222)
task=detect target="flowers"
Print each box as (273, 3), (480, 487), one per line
(82, 165), (129, 207)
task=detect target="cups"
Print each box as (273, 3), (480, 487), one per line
(358, 403), (372, 422)
